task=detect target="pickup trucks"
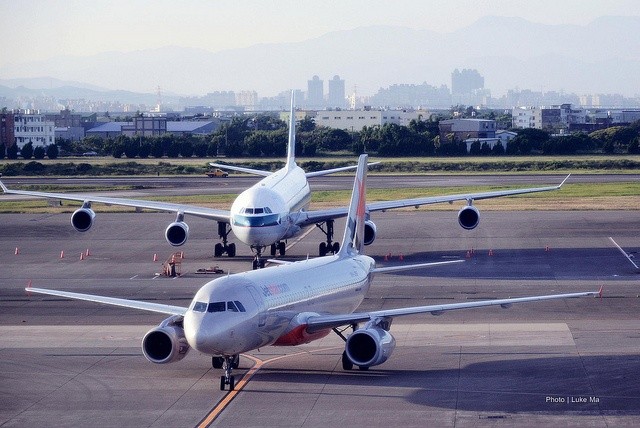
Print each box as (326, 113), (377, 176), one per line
(205, 169), (228, 178)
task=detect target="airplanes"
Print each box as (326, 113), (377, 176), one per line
(0, 89), (572, 270)
(24, 155), (601, 391)
